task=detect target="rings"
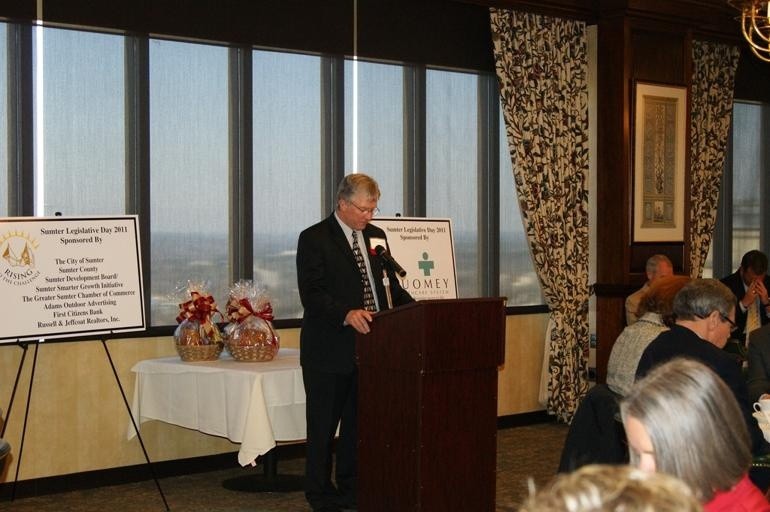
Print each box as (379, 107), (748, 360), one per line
(752, 290), (756, 293)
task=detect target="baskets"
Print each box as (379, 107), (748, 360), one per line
(227, 319), (280, 362)
(175, 320), (225, 362)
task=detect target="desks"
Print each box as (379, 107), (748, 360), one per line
(134, 347), (306, 492)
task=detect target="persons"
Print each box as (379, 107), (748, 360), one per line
(297, 174), (417, 510)
(747, 326), (770, 405)
(719, 252), (769, 331)
(625, 255), (675, 324)
(618, 356), (770, 512)
(606, 276), (691, 397)
(634, 278), (770, 460)
(520, 466), (702, 512)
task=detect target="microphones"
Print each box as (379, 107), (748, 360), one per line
(375, 245), (407, 277)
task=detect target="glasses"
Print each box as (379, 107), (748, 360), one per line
(349, 201), (380, 217)
(719, 312), (738, 333)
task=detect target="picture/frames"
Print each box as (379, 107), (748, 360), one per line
(630, 76), (690, 248)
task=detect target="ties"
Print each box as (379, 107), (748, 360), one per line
(746, 298), (758, 333)
(351, 230), (377, 312)
